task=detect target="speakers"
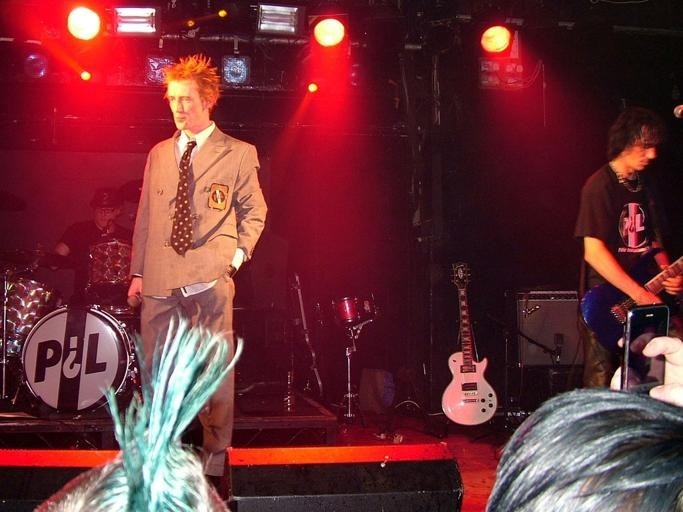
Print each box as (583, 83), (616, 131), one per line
(517, 299), (584, 366)
(0, 447), (122, 512)
(221, 441), (466, 512)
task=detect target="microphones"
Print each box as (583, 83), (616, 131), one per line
(127, 292), (142, 307)
(674, 105), (683, 118)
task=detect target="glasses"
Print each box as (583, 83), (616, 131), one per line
(633, 142), (654, 149)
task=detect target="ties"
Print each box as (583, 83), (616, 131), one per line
(170, 142), (198, 255)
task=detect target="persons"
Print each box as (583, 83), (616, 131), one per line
(44, 186), (135, 261)
(568, 104), (683, 394)
(27, 314), (245, 511)
(480, 386), (683, 511)
(122, 53), (273, 492)
(606, 334), (683, 410)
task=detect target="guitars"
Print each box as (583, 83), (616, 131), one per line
(581, 248), (683, 352)
(441, 261), (497, 426)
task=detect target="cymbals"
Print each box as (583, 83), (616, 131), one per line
(113, 209), (138, 232)
(119, 179), (143, 204)
(0, 248), (75, 270)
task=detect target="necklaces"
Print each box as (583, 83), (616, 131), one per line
(613, 169), (642, 196)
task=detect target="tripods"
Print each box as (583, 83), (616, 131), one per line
(335, 331), (368, 428)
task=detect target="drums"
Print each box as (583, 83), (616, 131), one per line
(96, 282), (140, 320)
(313, 293), (384, 330)
(89, 236), (132, 289)
(0, 275), (64, 360)
(20, 304), (139, 420)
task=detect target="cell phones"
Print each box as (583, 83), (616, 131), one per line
(622, 303), (671, 393)
